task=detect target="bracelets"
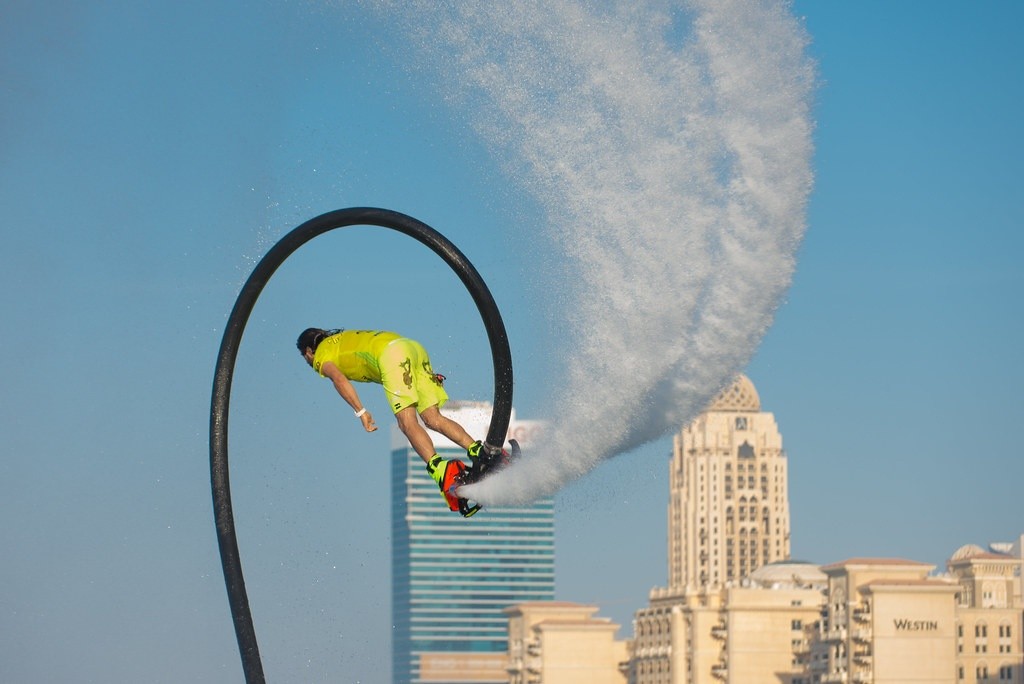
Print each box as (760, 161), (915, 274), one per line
(354, 408), (366, 418)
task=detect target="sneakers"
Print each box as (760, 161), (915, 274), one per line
(426, 453), (466, 510)
(467, 441), (510, 472)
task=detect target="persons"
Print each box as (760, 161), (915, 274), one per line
(297, 328), (511, 512)
